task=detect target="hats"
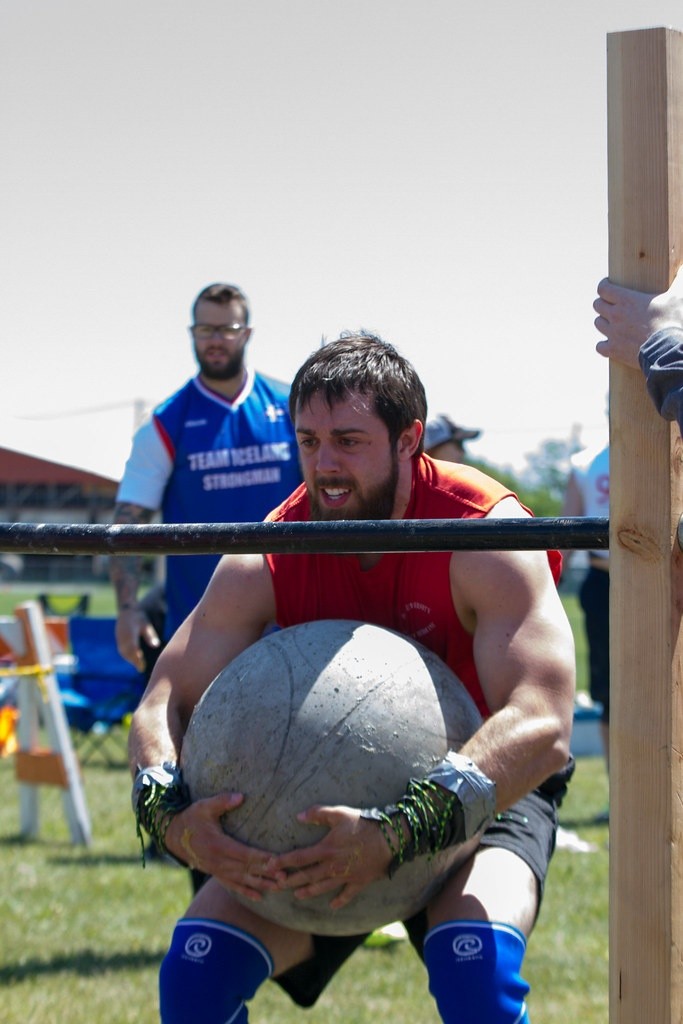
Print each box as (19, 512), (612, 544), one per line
(422, 413), (480, 455)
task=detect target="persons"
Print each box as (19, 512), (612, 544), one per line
(114, 284), (304, 672)
(420, 411), (481, 463)
(125, 333), (577, 1022)
(593, 260), (683, 440)
(576, 442), (611, 773)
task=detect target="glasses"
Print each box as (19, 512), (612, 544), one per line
(188, 321), (248, 340)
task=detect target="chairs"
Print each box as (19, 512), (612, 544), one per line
(50, 612), (150, 771)
